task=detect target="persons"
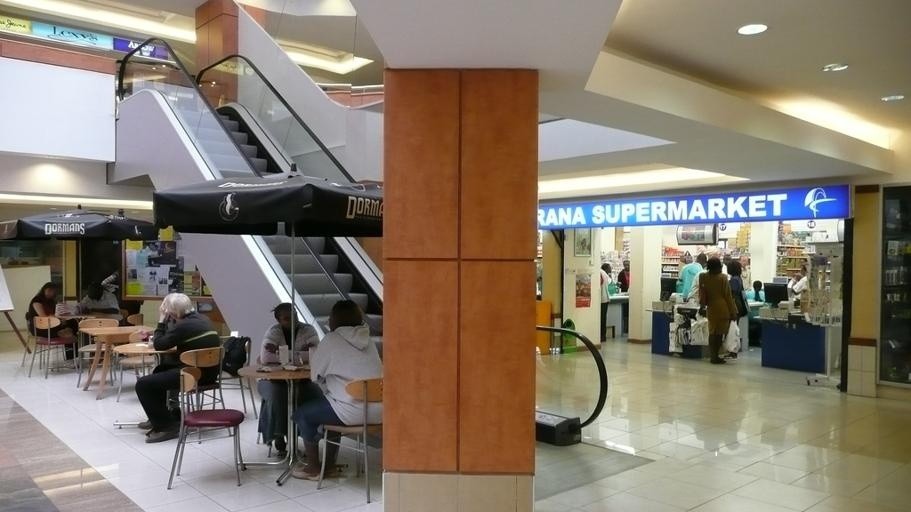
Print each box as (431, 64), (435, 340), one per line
(617, 260), (630, 334)
(675, 253), (765, 364)
(258, 303), (321, 457)
(27, 281), (80, 370)
(134, 293), (221, 444)
(100, 264), (144, 357)
(600, 268), (611, 342)
(602, 263), (612, 284)
(291, 300), (384, 481)
(787, 257), (809, 300)
(74, 281), (125, 365)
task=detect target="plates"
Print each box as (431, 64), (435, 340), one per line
(282, 366), (305, 371)
(302, 367), (310, 370)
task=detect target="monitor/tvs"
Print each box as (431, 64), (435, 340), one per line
(661, 278), (682, 301)
(773, 276), (788, 286)
(764, 283), (788, 308)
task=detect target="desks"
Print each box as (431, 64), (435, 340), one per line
(237, 361), (350, 486)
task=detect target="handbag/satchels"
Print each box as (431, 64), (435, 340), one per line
(56, 304), (78, 316)
(740, 290), (751, 313)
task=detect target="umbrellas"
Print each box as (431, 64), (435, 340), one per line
(1, 203), (182, 375)
(152, 162), (383, 469)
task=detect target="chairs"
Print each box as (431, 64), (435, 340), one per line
(165, 366), (247, 492)
(313, 374), (382, 505)
(252, 353), (278, 458)
(22, 298), (259, 431)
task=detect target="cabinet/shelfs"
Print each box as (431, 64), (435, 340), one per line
(601, 232), (630, 282)
(661, 244), (683, 280)
(692, 246), (750, 290)
(777, 230), (831, 290)
(877, 187), (911, 390)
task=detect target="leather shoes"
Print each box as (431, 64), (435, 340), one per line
(292, 467), (321, 480)
(138, 420), (153, 429)
(711, 359), (727, 364)
(145, 432), (179, 443)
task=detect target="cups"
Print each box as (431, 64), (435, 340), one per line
(274, 344), (290, 365)
(309, 346), (317, 368)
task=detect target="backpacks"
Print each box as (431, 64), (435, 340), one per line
(222, 337), (251, 377)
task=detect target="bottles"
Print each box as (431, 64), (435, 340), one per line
(146, 336), (154, 349)
(293, 352), (303, 366)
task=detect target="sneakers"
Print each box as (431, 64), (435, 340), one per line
(724, 353), (737, 359)
